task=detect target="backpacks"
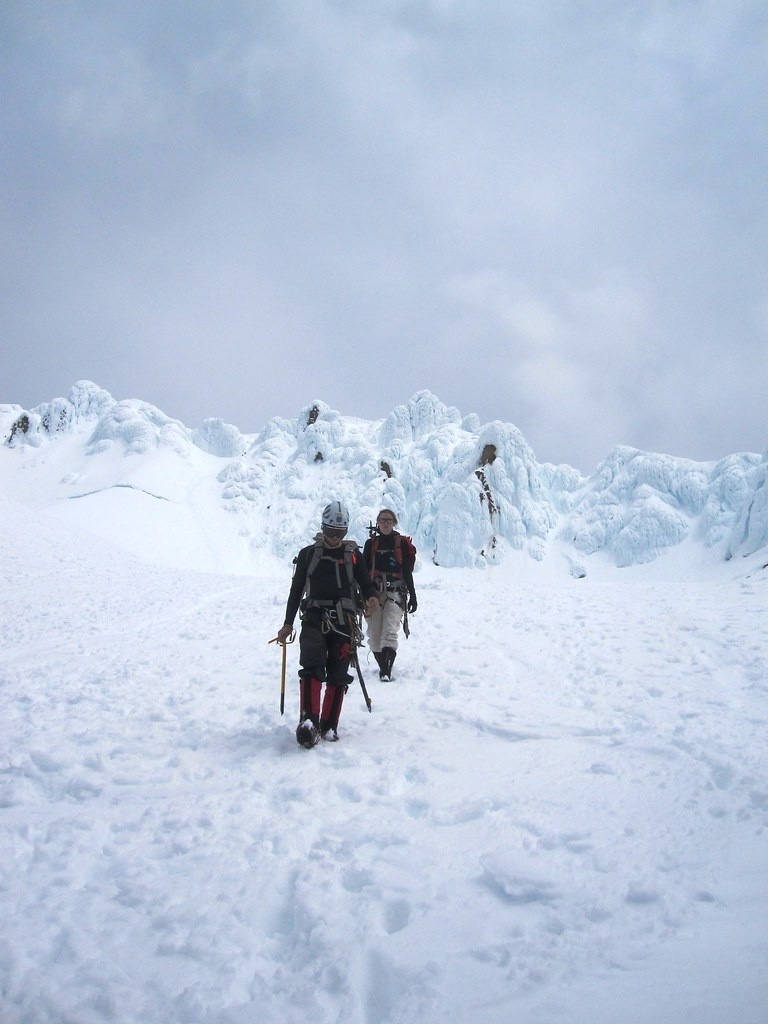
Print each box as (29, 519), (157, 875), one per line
(372, 530), (416, 573)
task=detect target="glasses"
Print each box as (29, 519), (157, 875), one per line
(320, 524), (347, 540)
(378, 517), (395, 524)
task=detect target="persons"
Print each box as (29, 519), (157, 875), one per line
(278, 502), (379, 750)
(363, 510), (417, 681)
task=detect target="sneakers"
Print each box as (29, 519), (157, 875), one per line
(319, 717), (338, 742)
(296, 713), (320, 750)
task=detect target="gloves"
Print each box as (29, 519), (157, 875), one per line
(278, 624), (293, 643)
(407, 594), (417, 615)
(367, 596), (379, 617)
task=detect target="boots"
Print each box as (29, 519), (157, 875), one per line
(371, 651), (383, 669)
(379, 647), (396, 681)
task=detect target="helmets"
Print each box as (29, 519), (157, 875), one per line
(322, 501), (350, 530)
